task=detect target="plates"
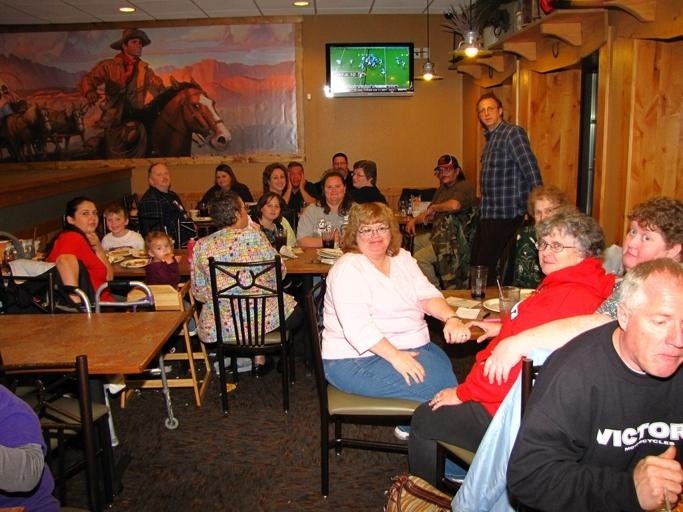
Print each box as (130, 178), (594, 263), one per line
(316, 248), (342, 259)
(515, 288), (537, 299)
(106, 248), (147, 270)
(482, 297), (500, 313)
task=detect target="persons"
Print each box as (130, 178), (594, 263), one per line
(407, 203), (615, 487)
(143, 232), (198, 337)
(467, 92), (543, 287)
(496, 183), (567, 289)
(407, 155), (477, 292)
(254, 153), (390, 305)
(1, 83), (19, 119)
(333, 49), (408, 77)
(482, 195), (681, 386)
(189, 190), (300, 378)
(504, 257), (681, 511)
(44, 197), (172, 376)
(80, 27), (165, 157)
(138, 162), (200, 250)
(320, 202), (471, 482)
(0, 381), (62, 512)
(198, 165), (255, 235)
(102, 204), (144, 252)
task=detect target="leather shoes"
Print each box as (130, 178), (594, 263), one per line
(251, 357), (275, 379)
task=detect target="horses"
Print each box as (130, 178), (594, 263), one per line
(0, 102), (86, 161)
(98, 74), (232, 157)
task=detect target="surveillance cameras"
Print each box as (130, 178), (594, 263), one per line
(444, 9), (456, 19)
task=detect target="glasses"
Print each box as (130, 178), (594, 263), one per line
(535, 239), (577, 252)
(356, 226), (390, 237)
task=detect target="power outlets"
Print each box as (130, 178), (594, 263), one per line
(413, 47), (421, 60)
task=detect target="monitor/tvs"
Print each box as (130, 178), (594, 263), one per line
(325, 42), (415, 97)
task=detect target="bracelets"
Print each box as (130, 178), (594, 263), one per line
(446, 316), (460, 320)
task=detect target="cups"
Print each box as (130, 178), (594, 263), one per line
(498, 286), (519, 317)
(320, 231), (334, 248)
(470, 266), (487, 298)
(187, 202), (211, 223)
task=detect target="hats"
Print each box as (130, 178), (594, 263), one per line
(433, 155), (458, 171)
(110, 29), (150, 50)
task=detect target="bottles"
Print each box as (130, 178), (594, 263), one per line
(399, 200), (420, 218)
(340, 215), (352, 247)
(185, 236), (196, 261)
(1, 240), (38, 265)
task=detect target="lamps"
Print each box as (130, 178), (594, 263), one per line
(413, 1), (445, 83)
(446, 1), (493, 58)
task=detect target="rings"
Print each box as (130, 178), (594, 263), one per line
(462, 335), (467, 337)
